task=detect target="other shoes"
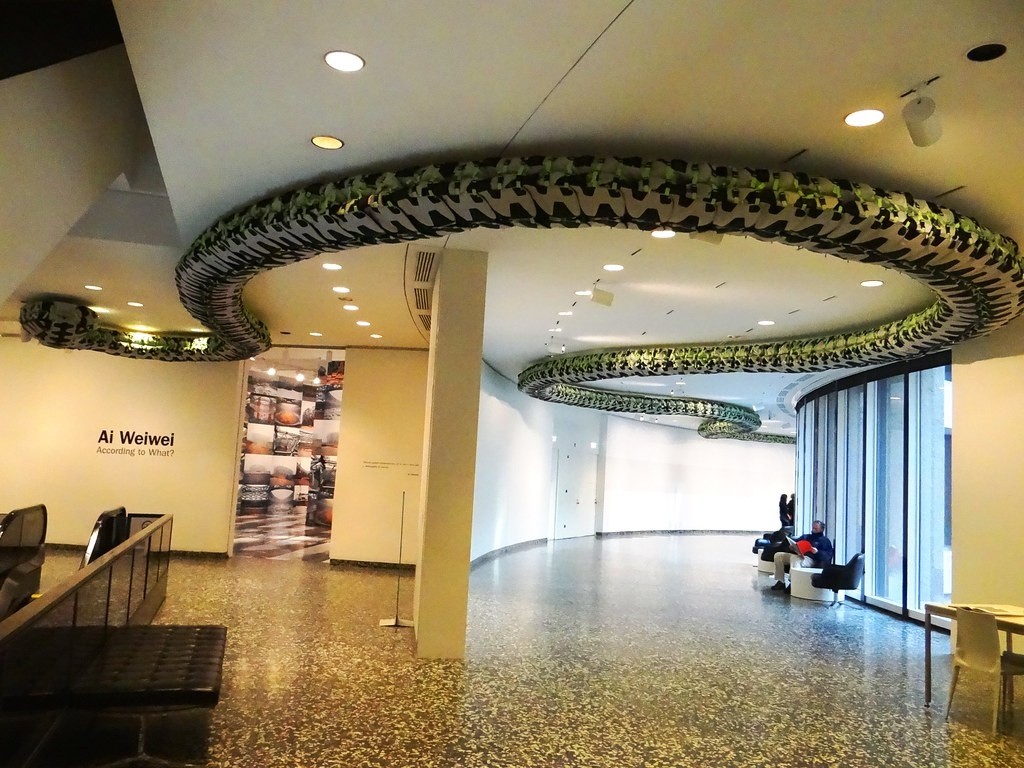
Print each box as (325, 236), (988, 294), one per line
(771, 581), (785, 590)
(784, 583), (791, 594)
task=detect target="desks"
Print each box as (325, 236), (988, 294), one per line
(921, 603), (1023, 708)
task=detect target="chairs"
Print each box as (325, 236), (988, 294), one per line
(77, 507), (128, 571)
(752, 525), (795, 567)
(783, 547), (834, 594)
(943, 607), (1023, 735)
(0, 503), (48, 617)
(810, 552), (865, 610)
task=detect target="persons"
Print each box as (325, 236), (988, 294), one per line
(779, 493), (792, 527)
(770, 519), (834, 594)
(788, 493), (795, 526)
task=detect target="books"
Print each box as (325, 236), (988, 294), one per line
(786, 535), (814, 560)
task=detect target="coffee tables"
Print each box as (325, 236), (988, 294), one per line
(790, 567), (845, 602)
(757, 548), (776, 575)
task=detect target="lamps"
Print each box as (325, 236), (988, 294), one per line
(313, 378), (319, 384)
(296, 373), (304, 382)
(902, 96), (942, 148)
(267, 367), (277, 376)
